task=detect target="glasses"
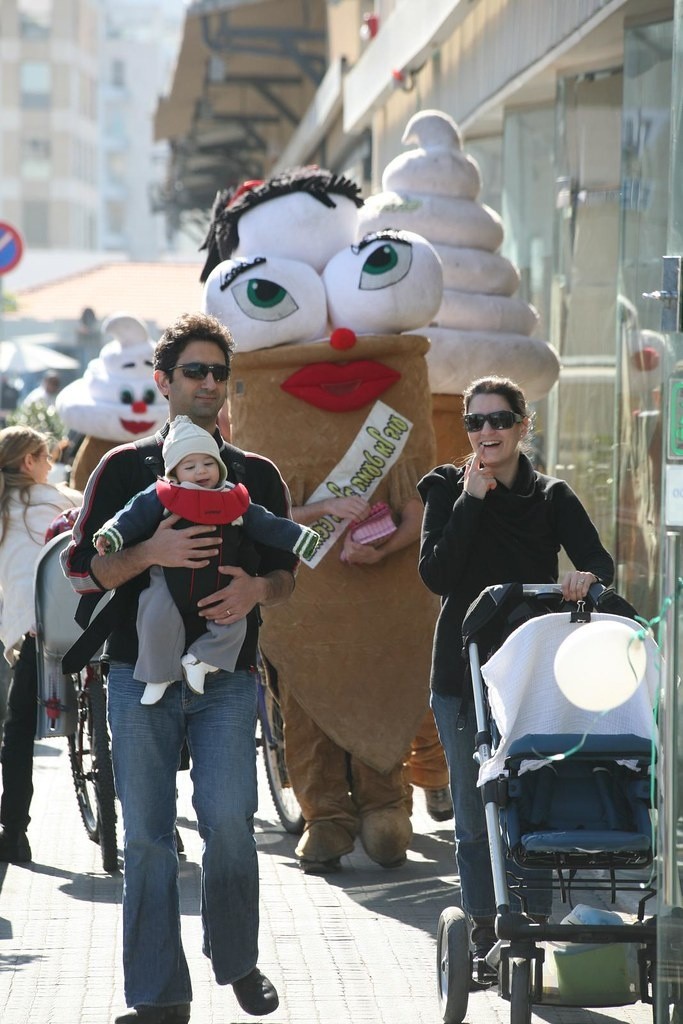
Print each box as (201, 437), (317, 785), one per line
(168, 362), (231, 381)
(463, 411), (523, 432)
(32, 454), (53, 463)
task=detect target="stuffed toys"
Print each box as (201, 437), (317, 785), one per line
(55, 316), (170, 494)
(354, 109), (560, 822)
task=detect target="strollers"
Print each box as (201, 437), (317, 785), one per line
(434, 581), (663, 1024)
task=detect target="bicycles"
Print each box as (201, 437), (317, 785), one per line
(254, 668), (311, 835)
(29, 527), (120, 873)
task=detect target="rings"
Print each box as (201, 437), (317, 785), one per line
(226, 610), (231, 616)
(576, 577), (585, 584)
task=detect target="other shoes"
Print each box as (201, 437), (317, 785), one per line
(202, 943), (279, 1016)
(424, 786), (454, 822)
(140, 680), (175, 705)
(0, 824), (31, 862)
(180, 653), (220, 695)
(115, 1004), (190, 1024)
(473, 944), (499, 984)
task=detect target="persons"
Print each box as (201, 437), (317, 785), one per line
(59, 313), (301, 1024)
(0, 308), (107, 863)
(416, 374), (614, 984)
(196, 165), (441, 873)
(91, 414), (320, 706)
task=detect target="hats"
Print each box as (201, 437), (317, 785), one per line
(163, 417), (228, 486)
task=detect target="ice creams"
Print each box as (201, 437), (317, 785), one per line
(345, 108), (560, 789)
